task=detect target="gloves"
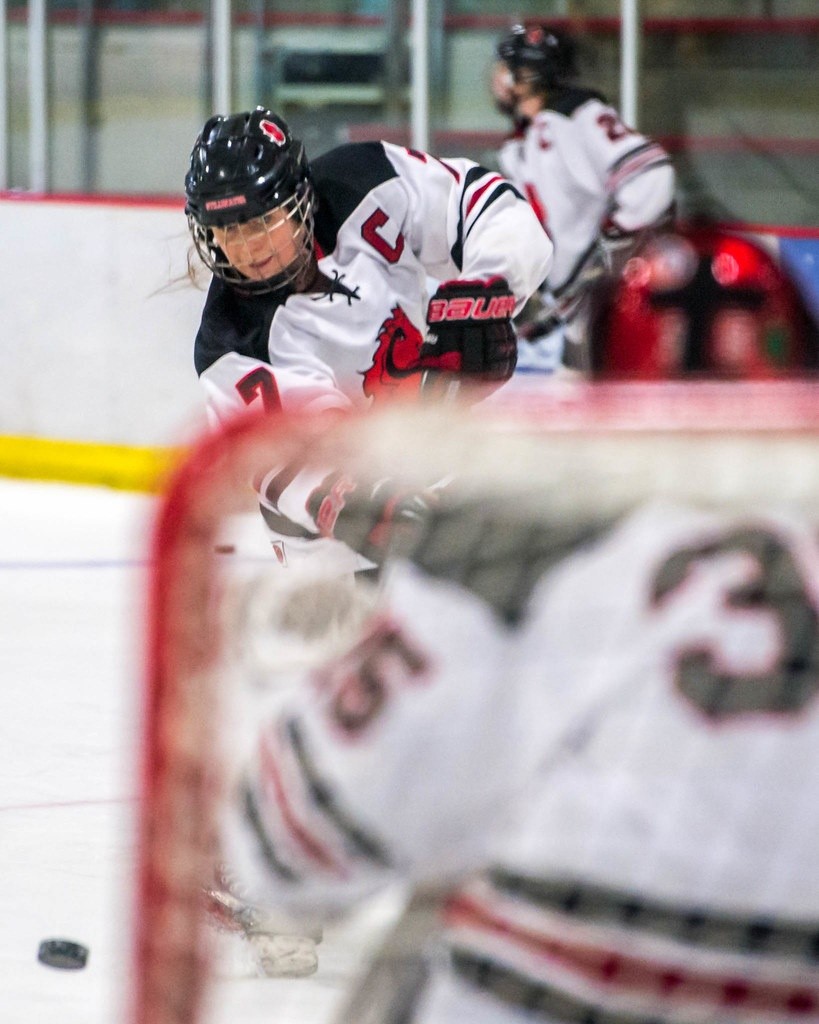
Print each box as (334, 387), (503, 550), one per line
(416, 275), (519, 407)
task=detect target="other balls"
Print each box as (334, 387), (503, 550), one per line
(37, 940), (90, 972)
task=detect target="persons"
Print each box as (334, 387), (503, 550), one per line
(206, 218), (819, 1024)
(186, 106), (554, 590)
(489, 23), (676, 377)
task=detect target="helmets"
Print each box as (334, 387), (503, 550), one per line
(184, 105), (317, 297)
(490, 23), (576, 116)
(593, 226), (804, 380)
